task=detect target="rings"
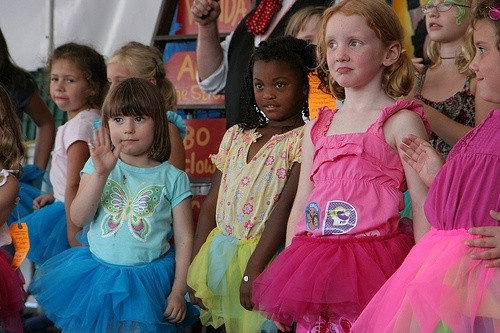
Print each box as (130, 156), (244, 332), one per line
(88, 144), (95, 149)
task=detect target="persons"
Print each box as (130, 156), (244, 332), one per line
(0, 28), (56, 170)
(399, 0), (500, 220)
(350, 0), (500, 333)
(27, 77), (193, 333)
(7, 43), (109, 292)
(191, 0), (335, 131)
(252, 0), (432, 333)
(0, 86), (27, 333)
(186, 35), (315, 333)
(92, 41), (186, 172)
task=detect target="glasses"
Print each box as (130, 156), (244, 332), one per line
(420, 1), (472, 15)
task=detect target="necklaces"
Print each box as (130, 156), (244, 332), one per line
(440, 57), (458, 59)
